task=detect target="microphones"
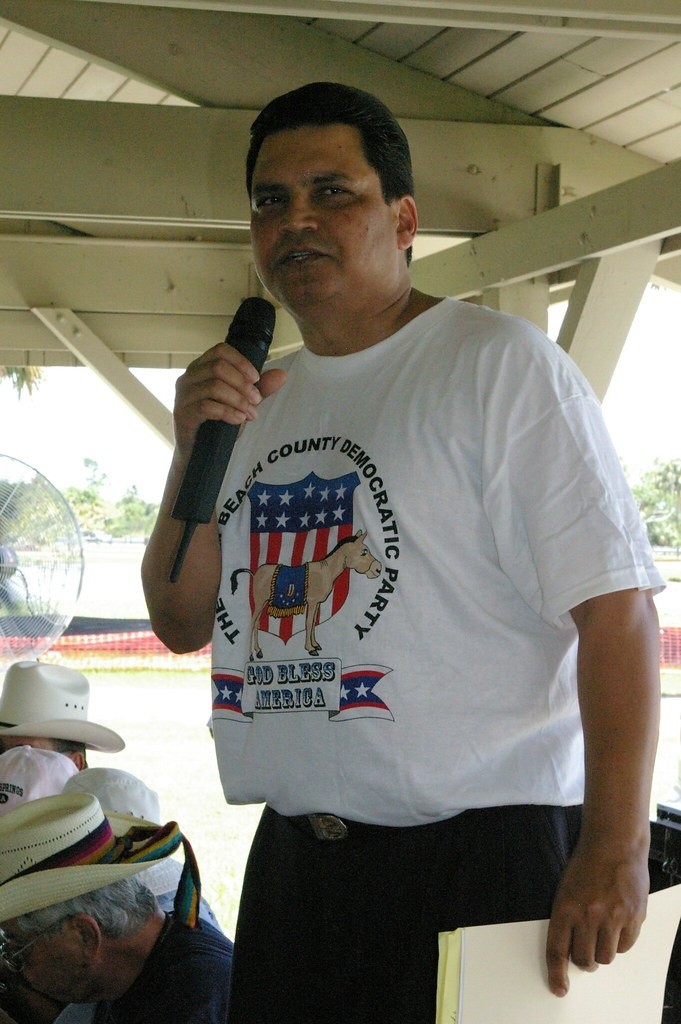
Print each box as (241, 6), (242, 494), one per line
(169, 297), (277, 583)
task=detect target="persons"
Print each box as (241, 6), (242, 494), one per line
(0, 661), (234, 1024)
(141, 81), (666, 1023)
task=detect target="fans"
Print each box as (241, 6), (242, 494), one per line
(0, 453), (86, 673)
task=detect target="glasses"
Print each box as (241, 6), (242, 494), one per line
(0, 922), (61, 972)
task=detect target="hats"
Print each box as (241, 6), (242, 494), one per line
(0, 661), (125, 753)
(62, 768), (160, 826)
(0, 792), (201, 930)
(0, 745), (80, 817)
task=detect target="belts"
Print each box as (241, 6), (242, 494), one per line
(286, 810), (406, 848)
